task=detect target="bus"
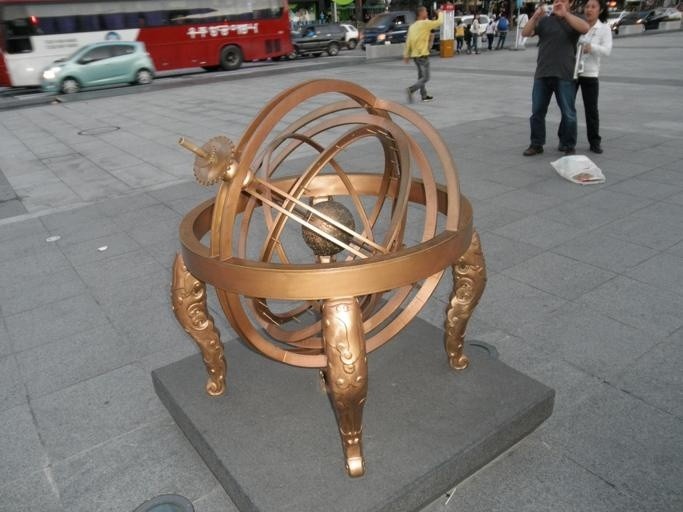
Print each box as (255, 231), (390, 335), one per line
(528, 0), (553, 16)
(0, 1), (287, 88)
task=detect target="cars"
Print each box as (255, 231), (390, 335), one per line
(338, 24), (359, 51)
(611, 8), (681, 35)
(38, 41), (155, 95)
(603, 10), (631, 31)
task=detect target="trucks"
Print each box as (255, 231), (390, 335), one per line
(358, 11), (440, 53)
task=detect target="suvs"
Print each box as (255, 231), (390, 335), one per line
(454, 15), (499, 39)
(272, 23), (346, 61)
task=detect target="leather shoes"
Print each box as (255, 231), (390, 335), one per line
(523, 144), (543, 155)
(565, 148), (575, 156)
(557, 141), (567, 151)
(590, 143), (602, 153)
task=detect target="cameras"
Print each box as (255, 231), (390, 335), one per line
(544, 5), (554, 12)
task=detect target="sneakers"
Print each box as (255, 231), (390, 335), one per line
(422, 96), (433, 101)
(405, 88), (413, 103)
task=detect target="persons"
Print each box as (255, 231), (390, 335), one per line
(521, 2), (589, 155)
(174, 12), (186, 24)
(136, 16), (145, 28)
(456, 12), (532, 54)
(574, 0), (613, 153)
(289, 13), (370, 33)
(402, 7), (444, 102)
(305, 28), (314, 36)
(394, 19), (402, 25)
